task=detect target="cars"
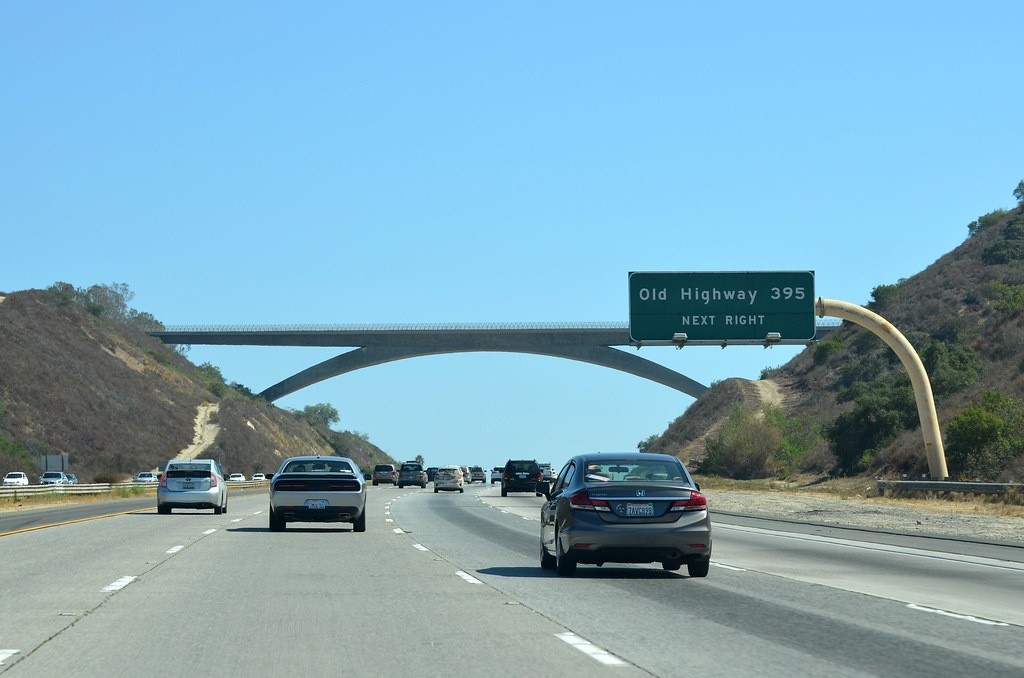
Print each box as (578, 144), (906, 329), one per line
(39, 471), (69, 485)
(65, 473), (78, 484)
(132, 472), (162, 483)
(265, 456), (371, 533)
(251, 473), (266, 481)
(461, 466), (472, 484)
(157, 457), (230, 515)
(536, 453), (713, 578)
(1, 471), (28, 486)
(228, 473), (245, 482)
(424, 468), (438, 481)
(435, 464), (464, 494)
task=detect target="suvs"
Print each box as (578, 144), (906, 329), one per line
(372, 464), (397, 486)
(501, 459), (543, 497)
(471, 465), (487, 483)
(552, 471), (558, 480)
(396, 460), (426, 489)
(490, 466), (505, 484)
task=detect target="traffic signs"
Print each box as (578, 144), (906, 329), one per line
(628, 270), (816, 346)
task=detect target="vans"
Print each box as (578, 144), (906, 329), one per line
(537, 462), (552, 483)
